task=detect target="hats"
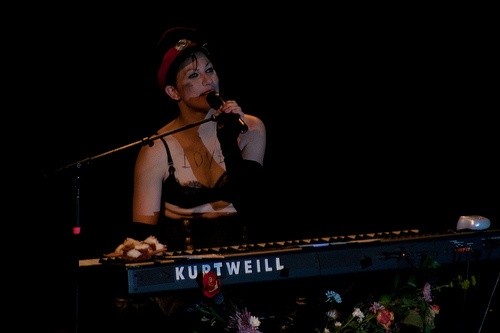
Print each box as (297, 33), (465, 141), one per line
(156, 37), (213, 97)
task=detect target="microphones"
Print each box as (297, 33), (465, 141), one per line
(206, 91), (248, 134)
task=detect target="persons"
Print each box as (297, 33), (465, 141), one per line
(133, 39), (265, 254)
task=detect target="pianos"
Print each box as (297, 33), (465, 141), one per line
(77, 228), (500, 295)
